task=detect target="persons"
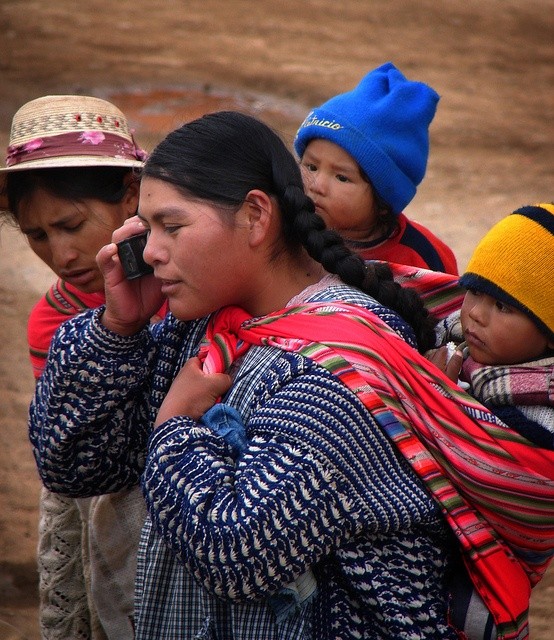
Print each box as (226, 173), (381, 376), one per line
(0, 94), (149, 639)
(294, 60), (458, 276)
(422, 202), (553, 451)
(27, 111), (455, 640)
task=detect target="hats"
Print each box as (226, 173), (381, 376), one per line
(0, 95), (145, 211)
(458, 203), (554, 338)
(295, 63), (440, 217)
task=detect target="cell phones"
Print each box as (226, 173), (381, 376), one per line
(113, 229), (154, 283)
